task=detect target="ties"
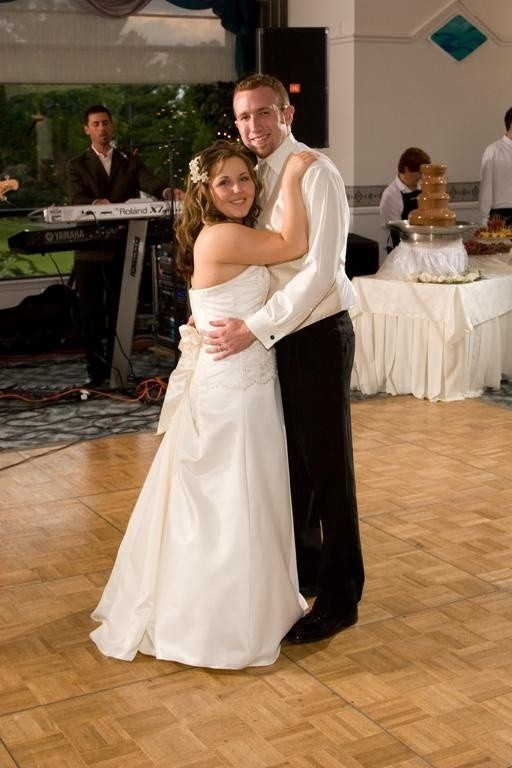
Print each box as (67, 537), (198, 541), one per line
(256, 162), (270, 199)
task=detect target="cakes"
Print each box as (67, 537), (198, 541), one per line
(408, 164), (457, 228)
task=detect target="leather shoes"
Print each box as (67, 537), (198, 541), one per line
(85, 365), (106, 388)
(288, 604), (359, 645)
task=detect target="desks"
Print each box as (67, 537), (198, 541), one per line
(350, 254), (512, 402)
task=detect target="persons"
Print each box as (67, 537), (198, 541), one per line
(189, 74), (363, 643)
(66, 104), (184, 392)
(476, 105), (512, 244)
(379, 147), (431, 258)
(86, 145), (318, 669)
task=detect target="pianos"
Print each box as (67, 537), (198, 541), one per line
(7, 201), (184, 255)
